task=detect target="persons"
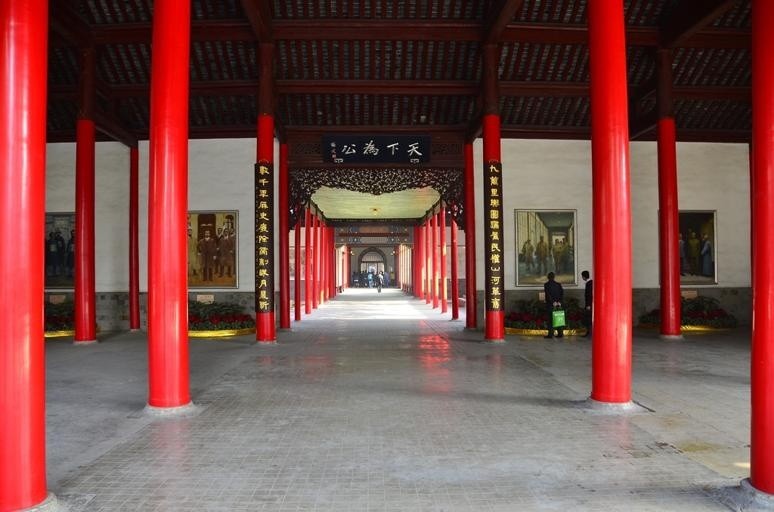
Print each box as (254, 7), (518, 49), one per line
(521, 232), (572, 282)
(679, 228), (713, 279)
(543, 272), (567, 340)
(188, 215), (236, 282)
(351, 267), (396, 294)
(46, 227), (76, 285)
(579, 271), (592, 337)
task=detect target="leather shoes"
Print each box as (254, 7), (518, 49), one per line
(544, 335), (551, 338)
(555, 334), (563, 338)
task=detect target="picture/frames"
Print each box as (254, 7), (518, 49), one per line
(513, 207), (578, 289)
(44, 211), (76, 293)
(656, 209), (720, 287)
(189, 208), (239, 289)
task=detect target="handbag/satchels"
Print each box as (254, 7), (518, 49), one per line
(551, 304), (566, 329)
